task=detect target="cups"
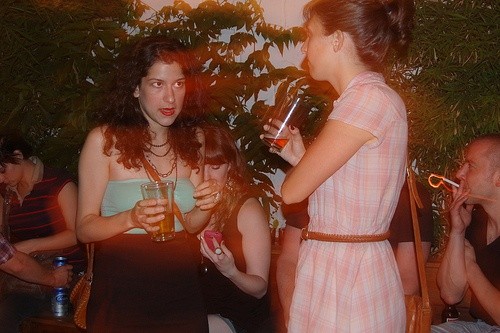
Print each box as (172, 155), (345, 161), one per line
(140, 180), (176, 240)
(261, 92), (311, 152)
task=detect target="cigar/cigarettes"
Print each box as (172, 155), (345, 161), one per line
(440, 176), (460, 189)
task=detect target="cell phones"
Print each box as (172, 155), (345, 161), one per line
(203, 231), (223, 252)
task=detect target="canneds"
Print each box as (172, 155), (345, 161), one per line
(53, 256), (69, 290)
(51, 289), (68, 316)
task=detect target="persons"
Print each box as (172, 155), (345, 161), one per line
(72, 33), (226, 333)
(187, 125), (275, 333)
(425, 133), (500, 333)
(274, 106), (436, 333)
(262, 0), (415, 333)
(0, 126), (89, 310)
(0, 231), (74, 289)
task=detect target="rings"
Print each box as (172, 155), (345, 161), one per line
(214, 247), (223, 256)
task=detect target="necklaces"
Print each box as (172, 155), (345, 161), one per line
(138, 129), (179, 192)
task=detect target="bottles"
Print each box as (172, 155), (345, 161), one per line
(440, 303), (460, 324)
(51, 257), (69, 320)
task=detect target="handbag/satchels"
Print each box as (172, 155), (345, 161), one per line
(69, 241), (98, 328)
(405, 293), (432, 333)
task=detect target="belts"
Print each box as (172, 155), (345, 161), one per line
(302, 227), (393, 241)
